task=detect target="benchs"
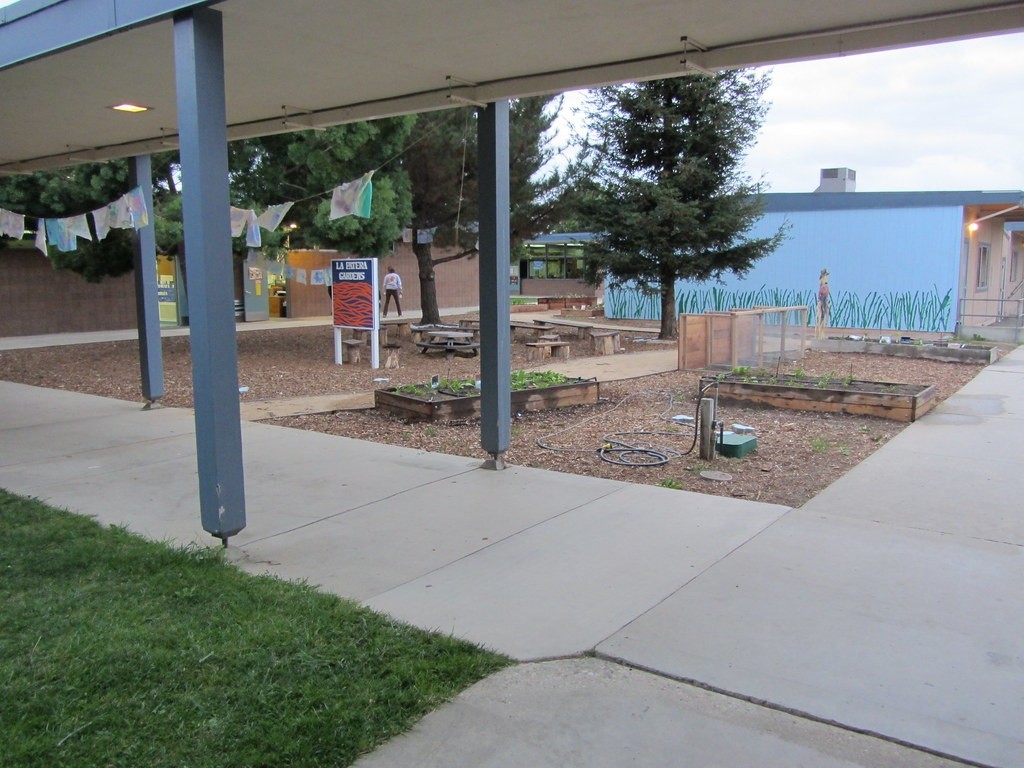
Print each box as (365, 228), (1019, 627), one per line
(411, 319), (480, 359)
(353, 327), (388, 344)
(532, 318), (593, 339)
(508, 324), (554, 343)
(376, 319), (410, 336)
(526, 343), (571, 360)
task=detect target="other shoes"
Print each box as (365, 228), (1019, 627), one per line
(398, 314), (402, 318)
(383, 314), (387, 318)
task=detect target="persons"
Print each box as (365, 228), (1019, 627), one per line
(815, 269), (829, 341)
(383, 266), (403, 318)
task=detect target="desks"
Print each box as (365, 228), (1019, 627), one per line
(427, 331), (473, 345)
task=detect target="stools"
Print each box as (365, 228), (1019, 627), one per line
(589, 331), (622, 355)
(382, 344), (401, 368)
(537, 334), (560, 342)
(344, 339), (364, 362)
